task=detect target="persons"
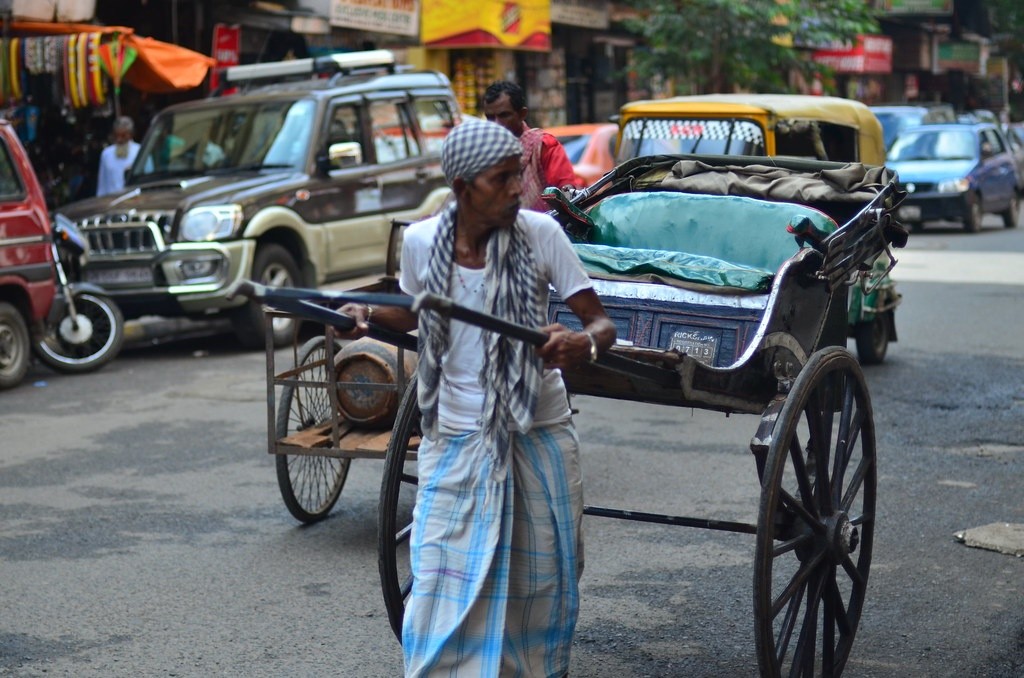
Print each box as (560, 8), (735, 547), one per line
(330, 122), (619, 678)
(475, 81), (588, 213)
(94, 115), (157, 195)
(317, 118), (351, 155)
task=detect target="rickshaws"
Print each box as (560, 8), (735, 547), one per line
(234, 154), (912, 677)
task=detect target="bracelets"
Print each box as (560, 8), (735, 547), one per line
(364, 301), (373, 324)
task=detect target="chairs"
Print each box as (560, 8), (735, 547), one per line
(540, 192), (841, 361)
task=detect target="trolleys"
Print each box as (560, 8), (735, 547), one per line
(263, 216), (425, 522)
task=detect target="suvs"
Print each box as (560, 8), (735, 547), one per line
(48, 51), (467, 350)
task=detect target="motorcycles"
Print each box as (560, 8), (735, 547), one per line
(615, 92), (902, 367)
(35, 211), (126, 374)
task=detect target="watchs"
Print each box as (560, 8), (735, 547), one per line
(582, 329), (598, 368)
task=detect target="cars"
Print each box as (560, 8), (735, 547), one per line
(543, 124), (618, 196)
(886, 123), (1022, 232)
